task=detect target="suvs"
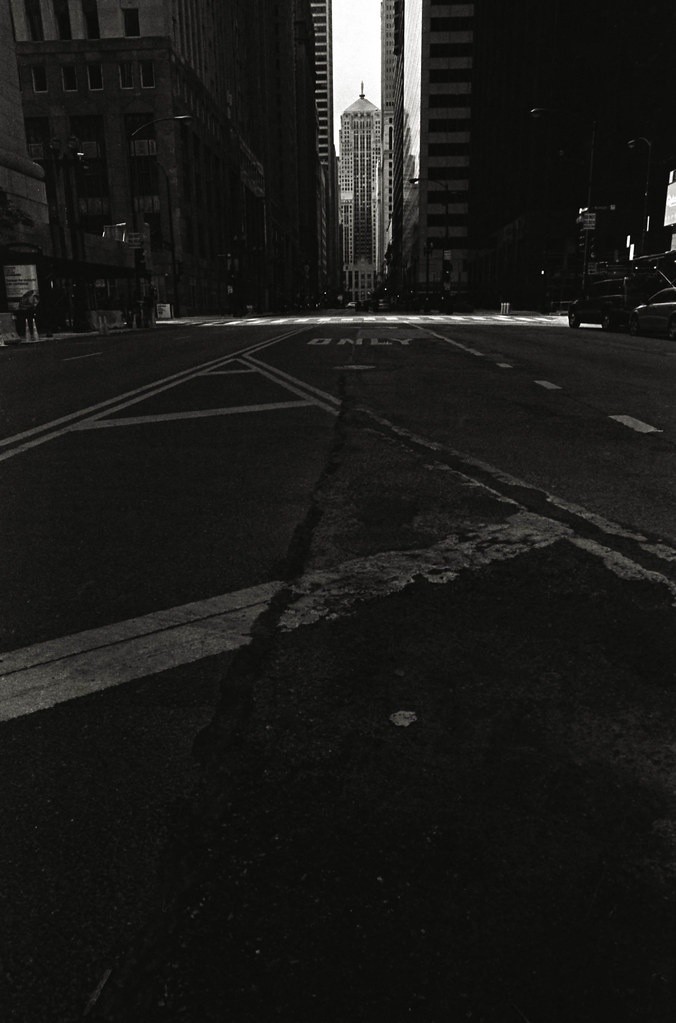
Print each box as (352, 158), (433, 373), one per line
(569, 274), (665, 331)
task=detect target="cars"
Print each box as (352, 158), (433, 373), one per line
(345, 299), (392, 312)
(628, 286), (676, 340)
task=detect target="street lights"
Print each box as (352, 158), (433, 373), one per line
(409, 178), (451, 314)
(128, 114), (193, 310)
(627, 135), (653, 257)
(530, 107), (598, 289)
(139, 153), (180, 316)
